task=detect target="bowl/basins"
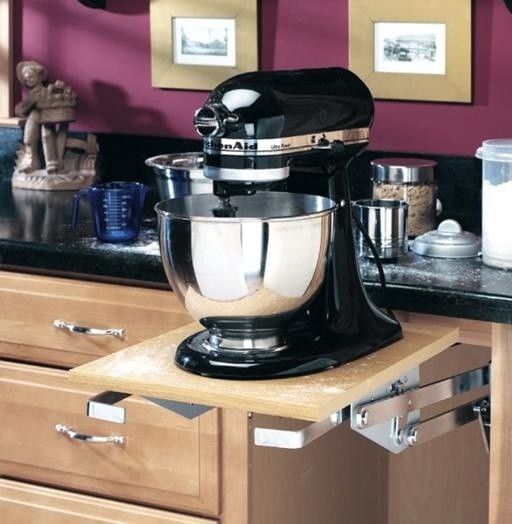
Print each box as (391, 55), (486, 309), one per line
(145, 149), (222, 199)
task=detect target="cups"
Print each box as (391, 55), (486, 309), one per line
(350, 195), (409, 259)
(69, 179), (151, 241)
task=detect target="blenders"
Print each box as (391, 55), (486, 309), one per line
(154, 63), (405, 379)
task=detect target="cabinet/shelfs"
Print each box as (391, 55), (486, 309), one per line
(0, 268), (252, 524)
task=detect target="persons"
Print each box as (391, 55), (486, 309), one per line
(15, 60), (77, 174)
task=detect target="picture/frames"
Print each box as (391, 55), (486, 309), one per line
(347, 0), (474, 105)
(149, 0), (260, 92)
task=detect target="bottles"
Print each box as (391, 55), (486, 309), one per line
(369, 156), (439, 241)
(474, 138), (511, 268)
